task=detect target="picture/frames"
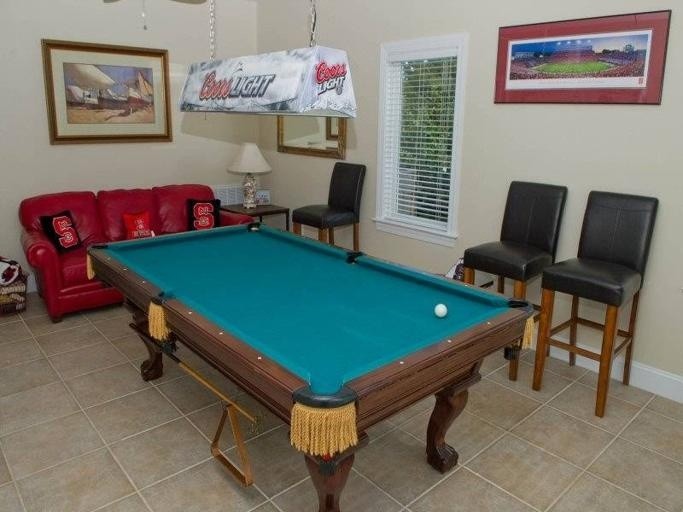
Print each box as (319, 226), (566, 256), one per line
(494, 9), (671, 105)
(40, 39), (173, 145)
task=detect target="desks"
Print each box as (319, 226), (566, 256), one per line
(87, 222), (534, 512)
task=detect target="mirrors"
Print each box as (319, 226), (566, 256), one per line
(277, 116), (347, 159)
(325, 117), (338, 140)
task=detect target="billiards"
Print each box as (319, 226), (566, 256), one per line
(435, 304), (447, 317)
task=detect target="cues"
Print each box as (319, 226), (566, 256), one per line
(129, 323), (257, 423)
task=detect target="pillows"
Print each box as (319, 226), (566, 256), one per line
(186, 199), (220, 231)
(40, 210), (82, 253)
(121, 210), (151, 239)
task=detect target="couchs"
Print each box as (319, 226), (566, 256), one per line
(18, 184), (254, 324)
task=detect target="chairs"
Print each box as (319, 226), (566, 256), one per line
(293, 162), (366, 251)
(464, 181), (568, 381)
(533, 191), (658, 417)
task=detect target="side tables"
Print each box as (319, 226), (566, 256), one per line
(221, 204), (291, 231)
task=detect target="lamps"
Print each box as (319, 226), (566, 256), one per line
(227, 143), (272, 210)
